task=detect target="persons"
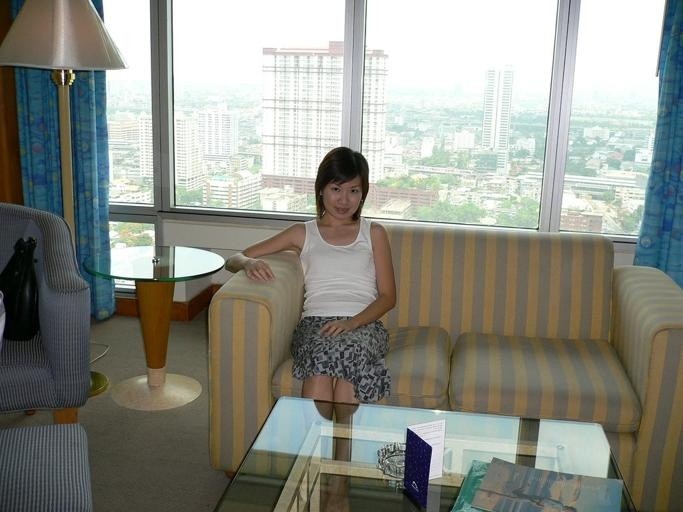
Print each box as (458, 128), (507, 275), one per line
(224, 146), (397, 511)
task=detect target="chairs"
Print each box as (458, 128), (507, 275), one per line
(0, 200), (91, 424)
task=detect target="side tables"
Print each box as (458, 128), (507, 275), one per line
(83, 244), (226, 412)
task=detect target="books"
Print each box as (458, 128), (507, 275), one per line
(404, 419), (445, 508)
(450, 456), (623, 512)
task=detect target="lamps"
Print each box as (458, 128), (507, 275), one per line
(0, 0), (127, 398)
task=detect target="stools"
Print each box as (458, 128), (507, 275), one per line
(1, 422), (94, 512)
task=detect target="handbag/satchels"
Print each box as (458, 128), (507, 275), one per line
(1, 236), (40, 342)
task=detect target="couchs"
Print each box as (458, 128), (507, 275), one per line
(208, 220), (683, 511)
